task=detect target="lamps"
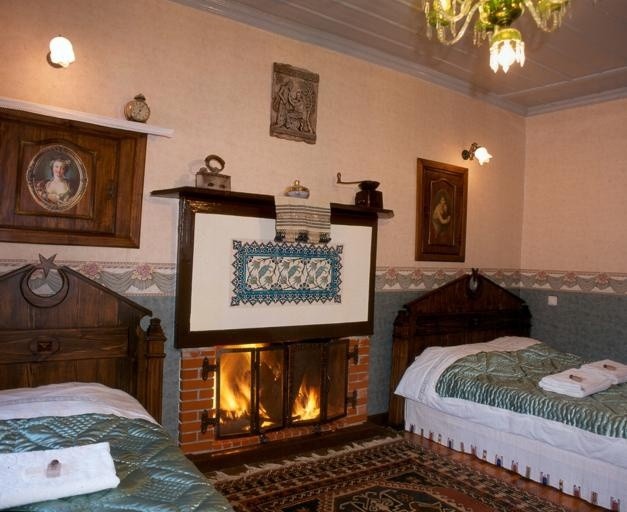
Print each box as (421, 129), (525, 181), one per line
(419, 2), (573, 73)
(46, 34), (77, 70)
(460, 141), (495, 167)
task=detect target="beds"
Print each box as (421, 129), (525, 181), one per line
(387, 271), (627, 512)
(0, 249), (239, 511)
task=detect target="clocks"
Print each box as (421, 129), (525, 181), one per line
(123, 93), (153, 121)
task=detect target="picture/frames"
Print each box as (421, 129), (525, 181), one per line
(417, 158), (468, 262)
(27, 145), (88, 212)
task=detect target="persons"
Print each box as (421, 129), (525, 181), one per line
(278, 80), (305, 128)
(36, 158), (78, 205)
(432, 196), (451, 240)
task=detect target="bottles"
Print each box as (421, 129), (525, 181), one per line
(285, 179), (310, 198)
(355, 181), (383, 209)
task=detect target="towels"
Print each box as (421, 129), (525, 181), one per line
(275, 196), (332, 245)
(538, 359), (627, 401)
(1, 442), (120, 510)
